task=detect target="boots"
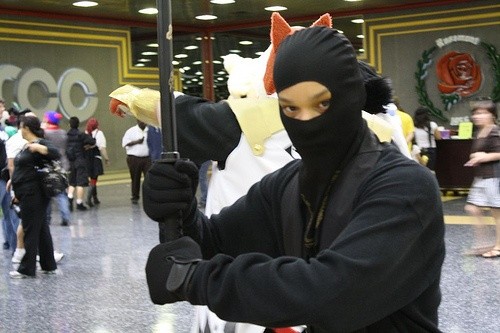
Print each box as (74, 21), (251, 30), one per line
(92, 187), (100, 204)
(86, 187), (96, 207)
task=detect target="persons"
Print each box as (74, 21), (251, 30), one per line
(142, 27), (446, 333)
(395, 98), (500, 258)
(0, 97), (211, 280)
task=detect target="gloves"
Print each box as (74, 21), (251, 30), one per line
(145, 236), (203, 305)
(142, 157), (199, 230)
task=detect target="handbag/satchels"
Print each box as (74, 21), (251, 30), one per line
(35, 141), (69, 197)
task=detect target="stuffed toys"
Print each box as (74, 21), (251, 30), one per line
(110, 13), (415, 333)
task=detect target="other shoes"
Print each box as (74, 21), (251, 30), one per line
(8, 271), (33, 279)
(131, 199), (138, 204)
(68, 199), (73, 212)
(36, 266), (57, 274)
(76, 203), (86, 210)
(12, 247), (25, 262)
(482, 249), (500, 258)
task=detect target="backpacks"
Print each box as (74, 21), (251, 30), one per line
(65, 132), (85, 161)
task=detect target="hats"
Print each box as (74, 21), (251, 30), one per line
(47, 112), (62, 124)
